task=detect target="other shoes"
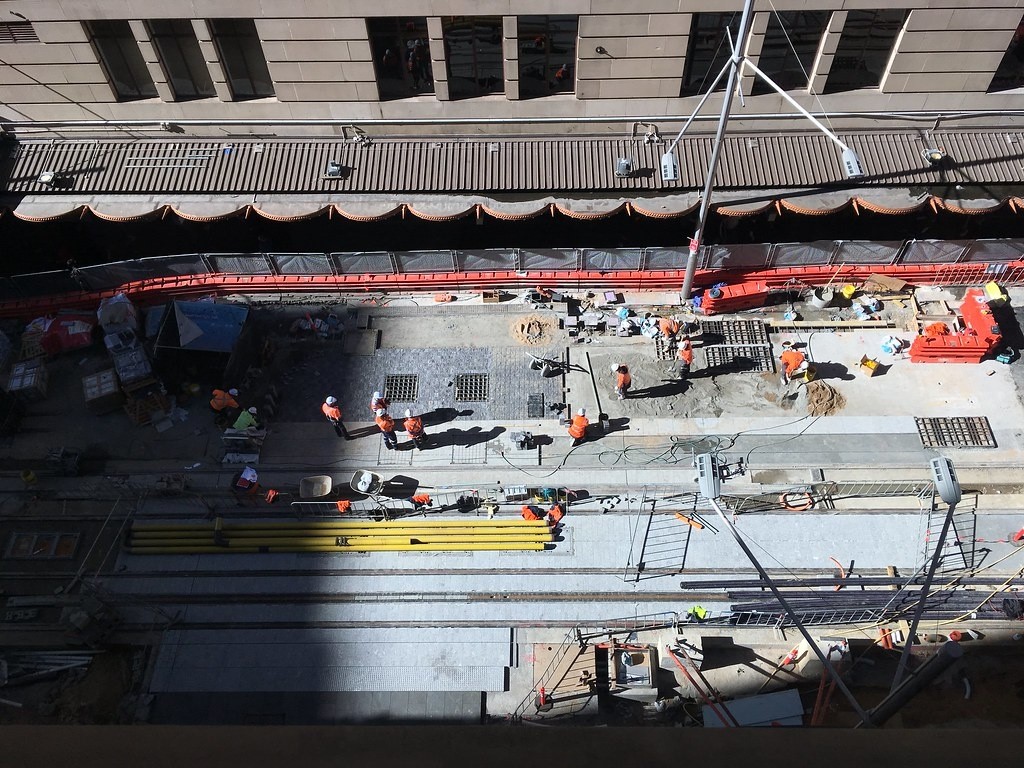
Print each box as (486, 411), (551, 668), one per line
(570, 438), (576, 446)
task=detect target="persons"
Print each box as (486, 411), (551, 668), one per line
(675, 339), (694, 377)
(374, 407), (398, 450)
(209, 387), (241, 414)
(371, 391), (388, 412)
(610, 363), (632, 401)
(568, 407), (589, 441)
(650, 317), (679, 353)
(233, 407), (263, 430)
(405, 408), (430, 451)
(779, 350), (810, 385)
(321, 396), (356, 441)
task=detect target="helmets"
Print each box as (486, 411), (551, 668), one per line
(374, 391), (383, 398)
(801, 361), (809, 369)
(229, 389), (238, 396)
(248, 407), (257, 414)
(405, 409), (413, 417)
(611, 364), (619, 371)
(578, 408), (586, 417)
(679, 341), (687, 350)
(376, 408), (386, 416)
(649, 318), (657, 327)
(326, 396), (337, 405)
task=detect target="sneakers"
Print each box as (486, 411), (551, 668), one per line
(780, 377), (786, 385)
(617, 395), (625, 400)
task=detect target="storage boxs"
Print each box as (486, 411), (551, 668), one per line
(3, 294), (167, 431)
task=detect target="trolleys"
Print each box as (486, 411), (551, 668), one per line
(350, 471), (404, 502)
(283, 475), (332, 499)
